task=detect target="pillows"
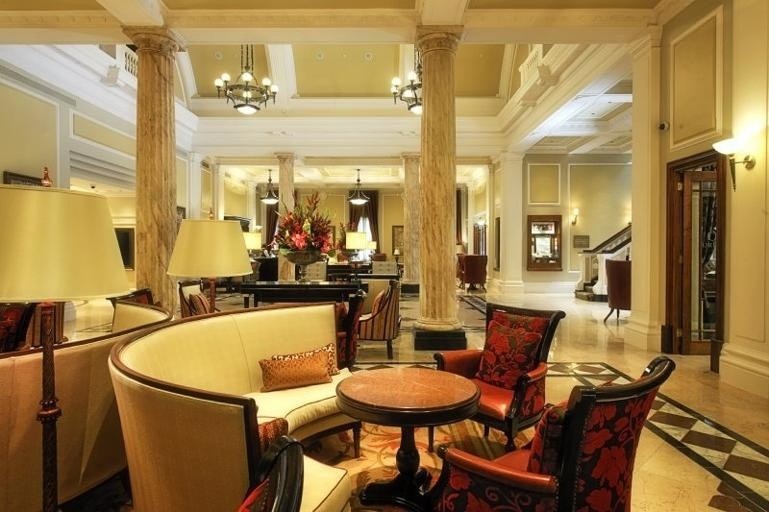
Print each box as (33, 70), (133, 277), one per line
(474, 319), (544, 394)
(257, 341), (340, 392)
(525, 379), (612, 475)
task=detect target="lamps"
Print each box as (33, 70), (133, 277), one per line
(713, 137), (756, 191)
(371, 241), (379, 259)
(258, 167), (280, 206)
(214, 43), (280, 118)
(243, 233), (262, 260)
(162, 218), (253, 316)
(0, 182), (138, 509)
(389, 44), (421, 115)
(346, 230), (370, 277)
(569, 206), (579, 227)
(347, 166), (371, 207)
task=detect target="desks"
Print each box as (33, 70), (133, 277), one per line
(334, 366), (482, 511)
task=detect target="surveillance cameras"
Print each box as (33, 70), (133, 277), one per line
(658, 122), (669, 131)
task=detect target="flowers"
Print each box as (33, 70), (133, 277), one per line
(264, 192), (345, 257)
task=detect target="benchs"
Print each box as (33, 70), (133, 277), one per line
(104, 293), (364, 511)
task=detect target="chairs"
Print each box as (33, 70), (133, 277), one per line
(426, 302), (566, 456)
(435, 352), (676, 511)
(174, 259), (410, 361)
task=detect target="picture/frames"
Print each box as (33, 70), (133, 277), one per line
(4, 172), (43, 187)
(113, 227), (134, 272)
(391, 223), (405, 257)
